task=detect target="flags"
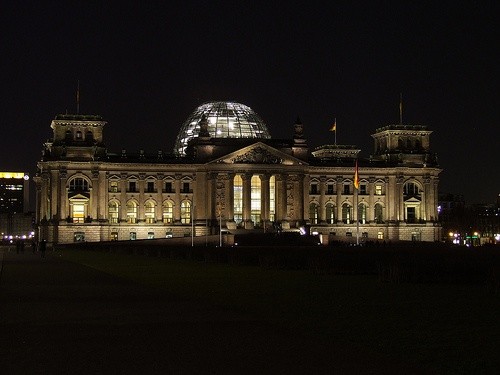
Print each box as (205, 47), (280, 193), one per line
(353, 161), (360, 194)
(329, 121), (336, 132)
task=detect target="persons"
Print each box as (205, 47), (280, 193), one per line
(16, 241), (20, 255)
(20, 241), (25, 254)
(32, 242), (37, 256)
(329, 239), (392, 249)
(40, 239), (47, 257)
